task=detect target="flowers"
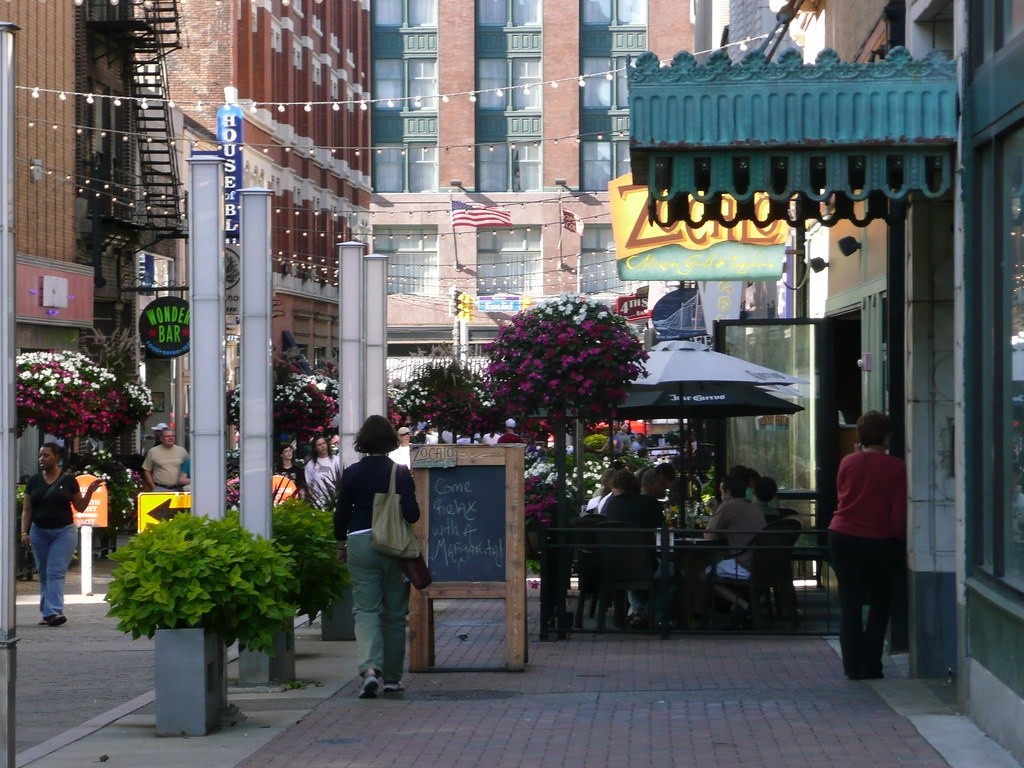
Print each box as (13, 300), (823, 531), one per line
(16, 351), (156, 443)
(225, 447), (241, 478)
(225, 362), (339, 442)
(523, 456), (618, 528)
(72, 449), (146, 527)
(481, 291), (651, 425)
(387, 362), (496, 435)
(227, 476), (240, 509)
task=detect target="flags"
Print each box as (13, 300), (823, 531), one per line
(452, 200), (512, 227)
(562, 207), (584, 236)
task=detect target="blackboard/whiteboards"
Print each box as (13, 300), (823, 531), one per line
(406, 444), (526, 599)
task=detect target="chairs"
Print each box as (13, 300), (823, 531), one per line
(567, 507), (838, 636)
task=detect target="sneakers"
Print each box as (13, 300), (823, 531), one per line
(358, 669), (379, 699)
(382, 680), (405, 692)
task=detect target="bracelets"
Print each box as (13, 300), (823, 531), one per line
(21, 533), (27, 537)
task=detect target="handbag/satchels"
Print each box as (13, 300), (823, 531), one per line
(394, 551), (433, 590)
(371, 491), (422, 559)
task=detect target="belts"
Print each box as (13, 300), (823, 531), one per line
(154, 482), (181, 490)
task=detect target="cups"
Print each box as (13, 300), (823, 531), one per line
(671, 519), (676, 527)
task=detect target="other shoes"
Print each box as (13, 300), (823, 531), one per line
(629, 614), (648, 629)
(47, 614), (66, 627)
(38, 618), (49, 625)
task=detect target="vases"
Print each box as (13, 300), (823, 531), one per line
(320, 588), (357, 642)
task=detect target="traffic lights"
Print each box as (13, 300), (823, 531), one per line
(521, 296), (533, 312)
(453, 290), (476, 322)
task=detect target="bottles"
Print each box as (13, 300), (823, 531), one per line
(663, 492), (717, 529)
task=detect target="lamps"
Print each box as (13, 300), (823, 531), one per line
(281, 262), (290, 276)
(811, 257), (829, 274)
(301, 271), (309, 282)
(555, 179), (566, 185)
(321, 276), (328, 286)
(451, 179), (463, 187)
(839, 235), (861, 257)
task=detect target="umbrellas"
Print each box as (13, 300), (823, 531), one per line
(609, 340), (810, 537)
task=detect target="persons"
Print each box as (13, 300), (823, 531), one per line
(586, 463), (676, 630)
(704, 464), (778, 630)
(273, 435), (340, 513)
(21, 442), (104, 627)
(334, 414), (420, 699)
(828, 409), (908, 681)
(387, 418), (522, 470)
(595, 424), (648, 462)
(141, 422), (190, 492)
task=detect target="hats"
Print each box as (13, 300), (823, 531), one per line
(150, 423), (168, 431)
(505, 418), (516, 428)
(397, 426), (410, 435)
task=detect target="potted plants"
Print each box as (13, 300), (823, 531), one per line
(227, 497), (352, 686)
(101, 508), (300, 740)
